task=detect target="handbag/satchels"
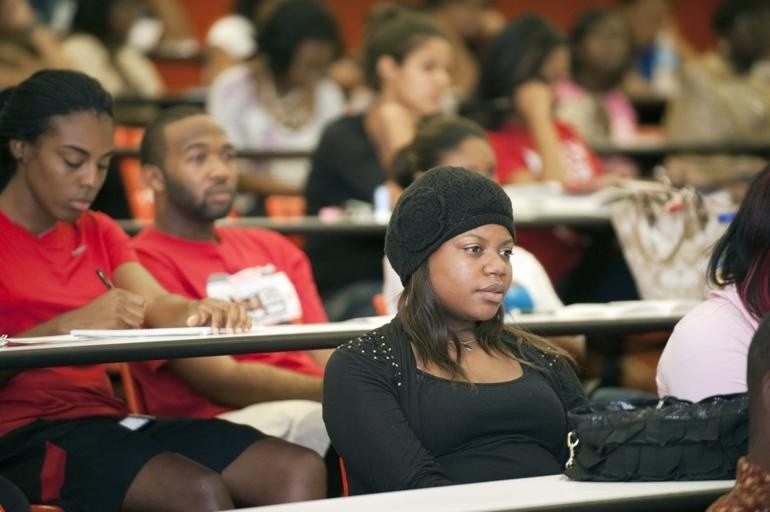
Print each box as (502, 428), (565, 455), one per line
(562, 391), (749, 481)
(611, 183), (718, 299)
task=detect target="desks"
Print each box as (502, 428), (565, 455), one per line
(0, 31), (769, 511)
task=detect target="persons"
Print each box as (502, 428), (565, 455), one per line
(320, 164), (596, 495)
(0, 70), (327, 512)
(1, 0), (769, 362)
(705, 308), (770, 512)
(654, 160), (770, 405)
(125, 102), (334, 460)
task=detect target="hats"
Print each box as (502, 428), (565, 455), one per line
(383, 166), (515, 282)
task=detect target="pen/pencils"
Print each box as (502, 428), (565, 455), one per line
(96, 269), (116, 289)
